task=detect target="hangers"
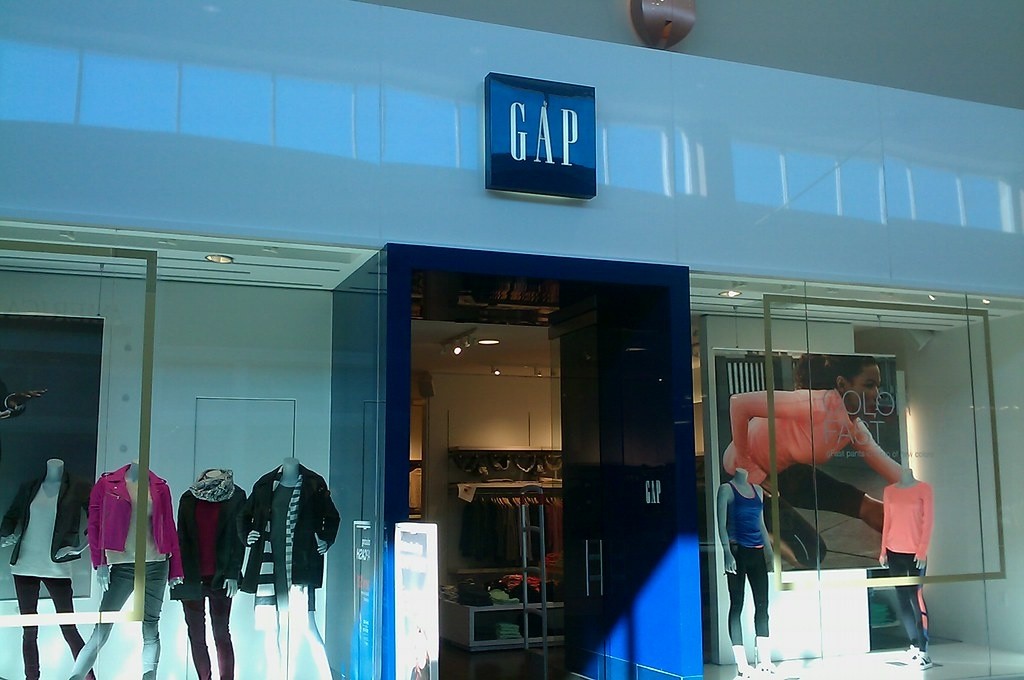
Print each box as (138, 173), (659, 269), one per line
(490, 496), (556, 508)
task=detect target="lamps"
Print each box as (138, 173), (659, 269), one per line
(438, 327), (476, 356)
(490, 365), (501, 376)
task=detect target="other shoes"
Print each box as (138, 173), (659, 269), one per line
(734, 665), (769, 679)
(755, 662), (788, 679)
(907, 644), (920, 659)
(910, 651), (932, 670)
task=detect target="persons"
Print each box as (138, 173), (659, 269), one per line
(719, 356), (903, 569)
(717, 468), (778, 678)
(0, 458), (341, 680)
(879, 468), (933, 670)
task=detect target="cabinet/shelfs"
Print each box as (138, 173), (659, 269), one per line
(439, 598), (565, 651)
(448, 446), (562, 573)
